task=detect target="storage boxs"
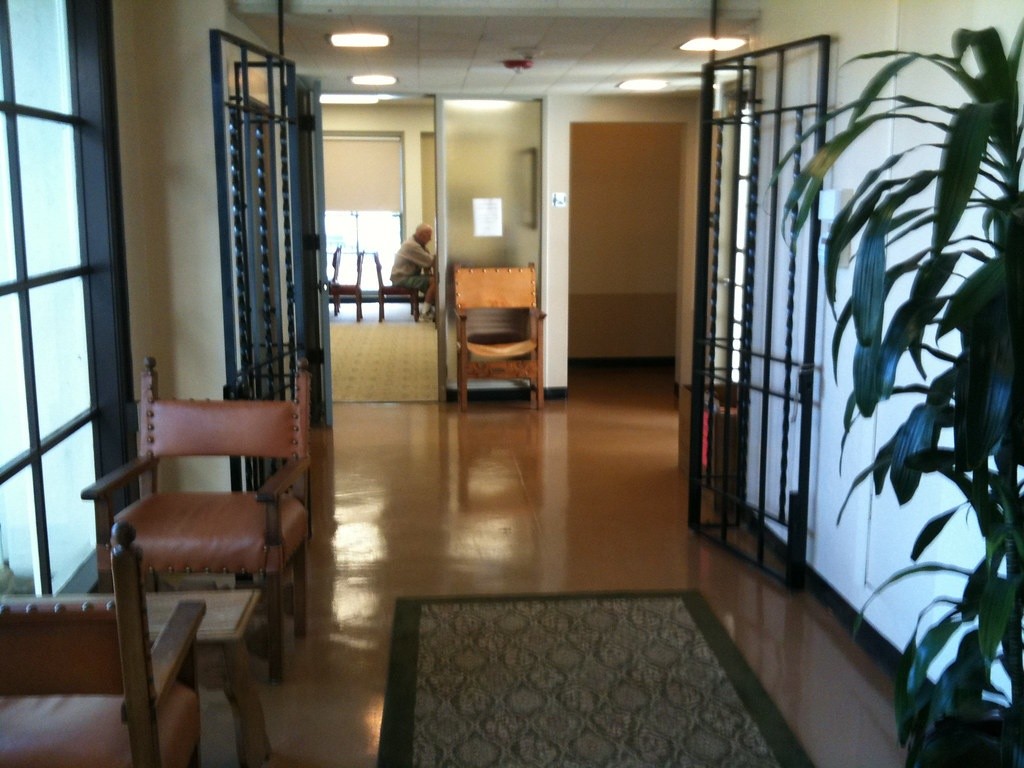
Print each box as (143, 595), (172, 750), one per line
(678, 383), (739, 488)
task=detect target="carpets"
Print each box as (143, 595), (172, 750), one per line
(376, 584), (819, 768)
(329, 321), (439, 403)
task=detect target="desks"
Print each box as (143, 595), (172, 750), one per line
(141, 590), (272, 768)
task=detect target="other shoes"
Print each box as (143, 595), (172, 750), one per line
(420, 308), (435, 315)
(418, 312), (433, 323)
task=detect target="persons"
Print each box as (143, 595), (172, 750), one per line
(390, 223), (435, 322)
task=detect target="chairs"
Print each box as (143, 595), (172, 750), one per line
(371, 251), (421, 323)
(335, 251), (365, 321)
(323, 244), (342, 318)
(0, 521), (204, 768)
(72, 351), (316, 687)
(454, 262), (546, 410)
